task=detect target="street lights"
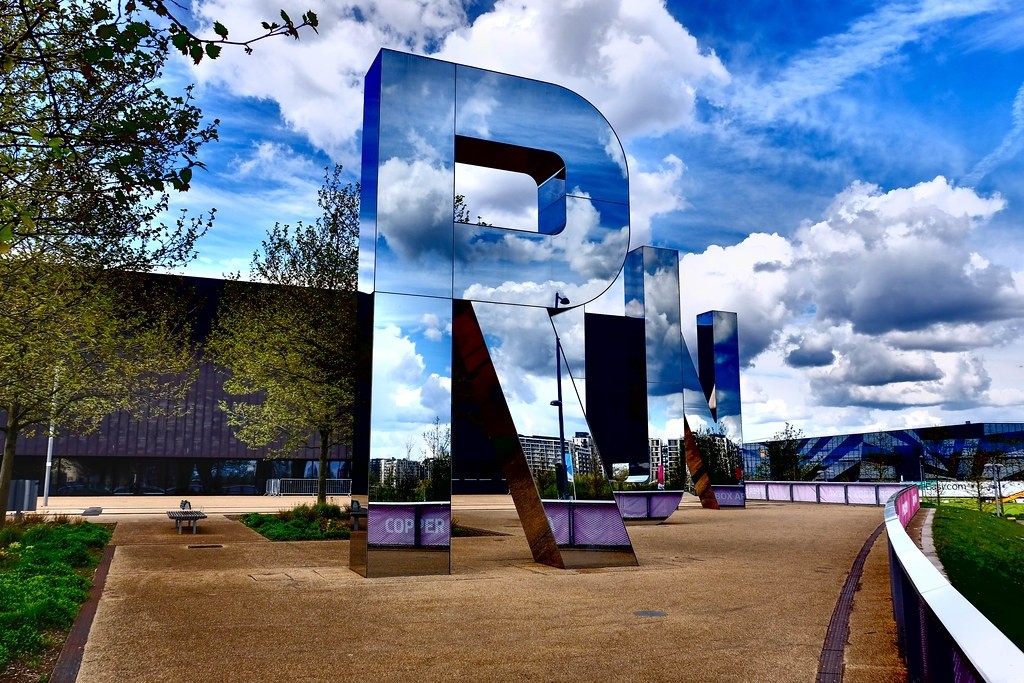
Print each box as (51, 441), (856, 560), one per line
(919, 455), (924, 507)
(984, 463), (1003, 517)
(549, 292), (572, 496)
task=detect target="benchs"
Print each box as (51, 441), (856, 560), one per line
(167, 500), (207, 535)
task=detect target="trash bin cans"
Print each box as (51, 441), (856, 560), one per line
(24, 480), (39, 511)
